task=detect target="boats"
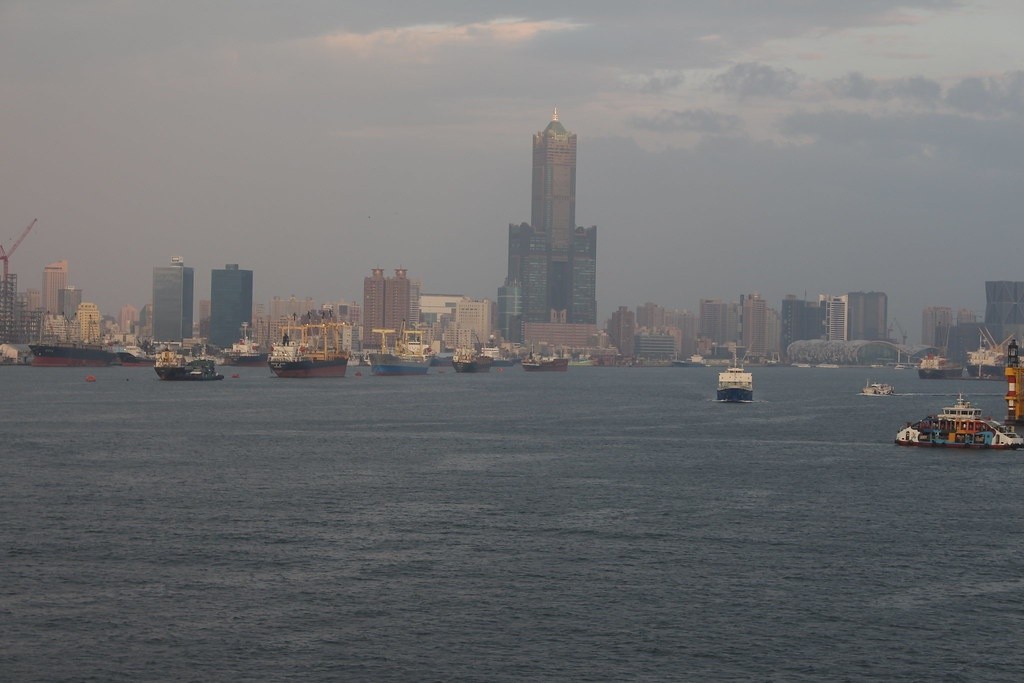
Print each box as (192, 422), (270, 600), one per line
(520, 352), (569, 371)
(451, 346), (516, 375)
(716, 346), (755, 402)
(671, 354), (707, 368)
(151, 350), (225, 381)
(367, 319), (434, 375)
(0, 344), (19, 366)
(964, 327), (1024, 378)
(267, 307), (352, 377)
(918, 323), (964, 380)
(28, 344), (153, 367)
(863, 378), (895, 395)
(223, 323), (261, 364)
(894, 393), (1024, 449)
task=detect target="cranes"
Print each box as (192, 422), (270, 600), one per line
(43, 309), (96, 343)
(0, 217), (39, 293)
